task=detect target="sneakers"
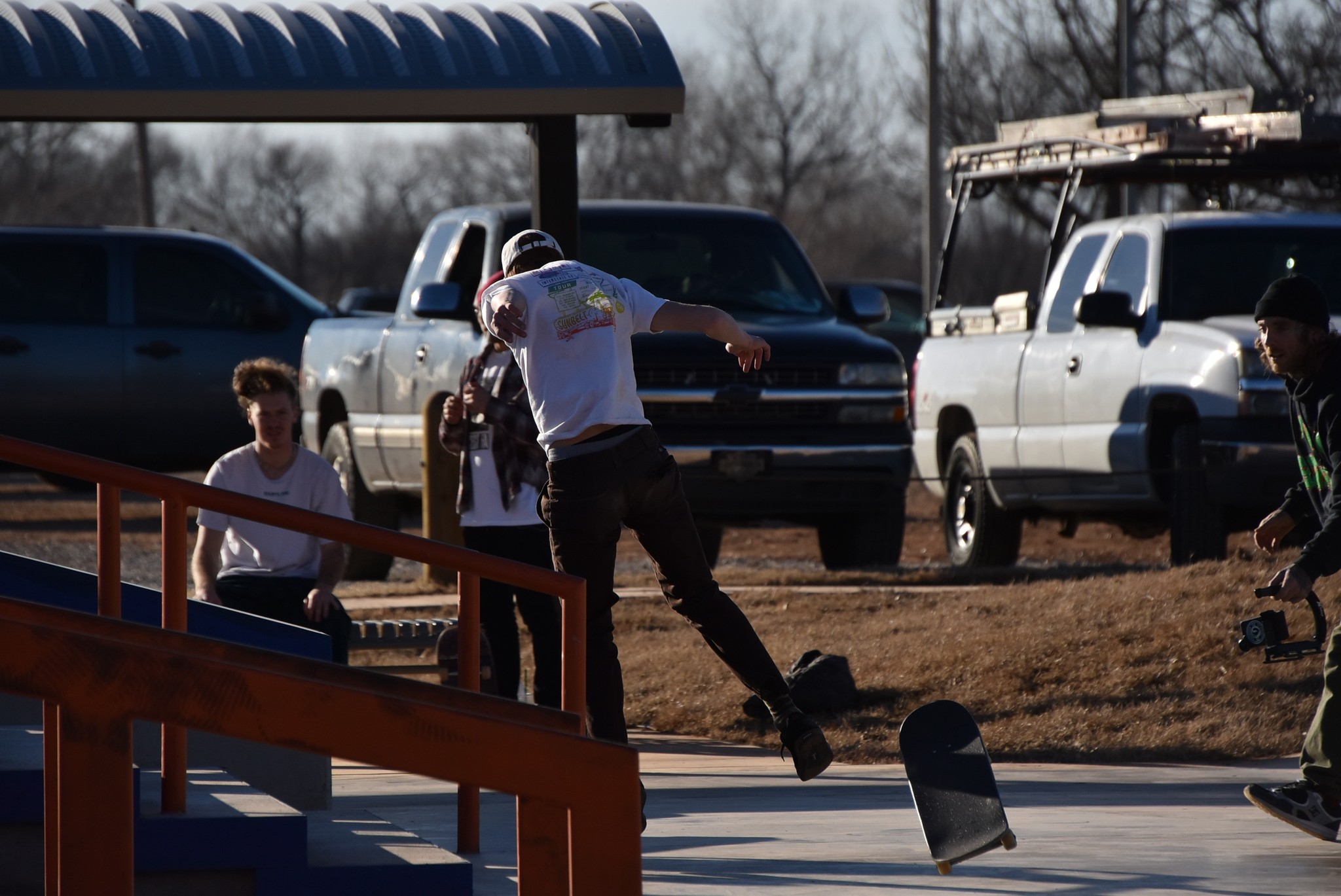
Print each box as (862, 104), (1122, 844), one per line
(1244, 778), (1341, 843)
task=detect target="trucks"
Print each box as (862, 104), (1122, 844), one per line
(826, 281), (931, 366)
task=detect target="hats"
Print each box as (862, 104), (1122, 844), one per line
(1253, 273), (1332, 331)
(501, 232), (566, 280)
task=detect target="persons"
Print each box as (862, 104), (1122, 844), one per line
(191, 357), (353, 668)
(480, 226), (834, 841)
(1243, 272), (1341, 840)
(439, 275), (562, 711)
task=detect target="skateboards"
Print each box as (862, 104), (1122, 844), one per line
(897, 698), (1018, 876)
(435, 621), (502, 698)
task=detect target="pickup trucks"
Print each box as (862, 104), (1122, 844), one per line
(914, 86), (1341, 587)
(300, 200), (914, 583)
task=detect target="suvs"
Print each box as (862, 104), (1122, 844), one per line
(0, 225), (344, 491)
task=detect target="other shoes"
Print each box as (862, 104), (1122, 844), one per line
(776, 713), (834, 783)
